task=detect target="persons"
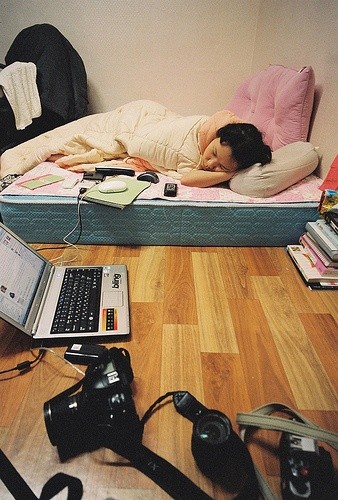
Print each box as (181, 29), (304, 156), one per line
(181, 110), (272, 188)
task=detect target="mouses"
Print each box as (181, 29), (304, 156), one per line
(99, 181), (128, 193)
(137, 172), (159, 184)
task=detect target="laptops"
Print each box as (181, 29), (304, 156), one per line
(0, 222), (131, 340)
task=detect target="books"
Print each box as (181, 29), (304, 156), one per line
(286, 212), (338, 291)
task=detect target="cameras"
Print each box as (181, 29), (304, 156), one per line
(192, 409), (250, 491)
(43, 347), (142, 447)
(278, 417), (333, 500)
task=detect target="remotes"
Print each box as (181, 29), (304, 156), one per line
(164, 183), (177, 196)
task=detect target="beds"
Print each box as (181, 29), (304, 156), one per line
(0, 161), (324, 247)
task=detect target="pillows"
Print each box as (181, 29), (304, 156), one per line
(229, 141), (322, 197)
